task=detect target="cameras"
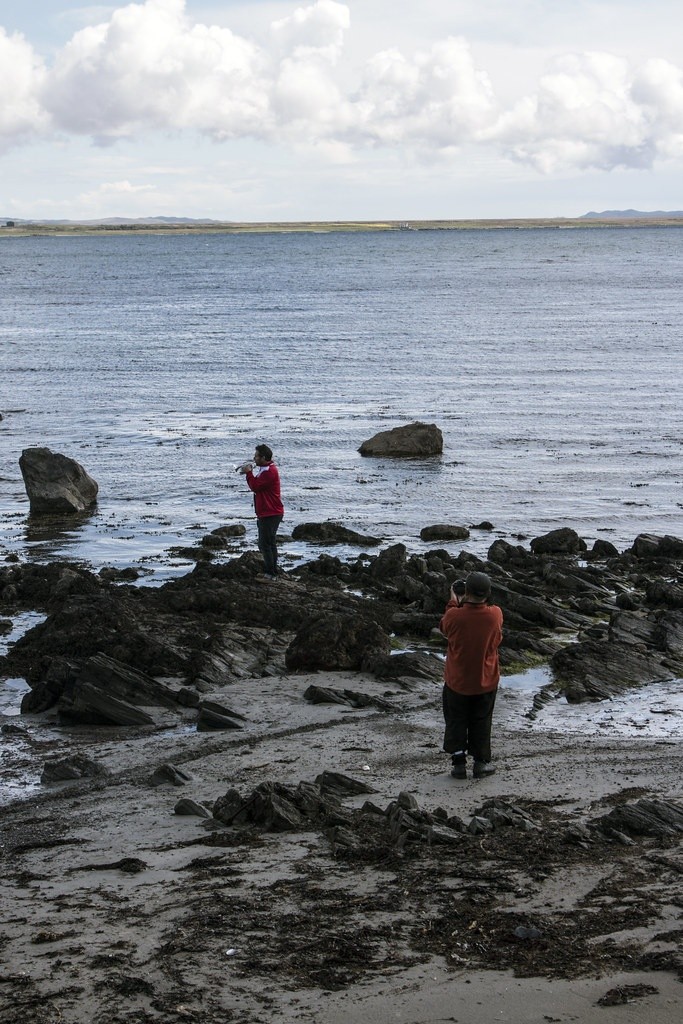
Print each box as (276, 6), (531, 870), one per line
(453, 581), (466, 596)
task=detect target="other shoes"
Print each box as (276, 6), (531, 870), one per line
(253, 571), (281, 586)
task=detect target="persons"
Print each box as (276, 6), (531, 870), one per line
(243, 444), (284, 575)
(440, 572), (503, 779)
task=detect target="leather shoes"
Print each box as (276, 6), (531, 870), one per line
(451, 765), (468, 779)
(473, 762), (495, 779)
